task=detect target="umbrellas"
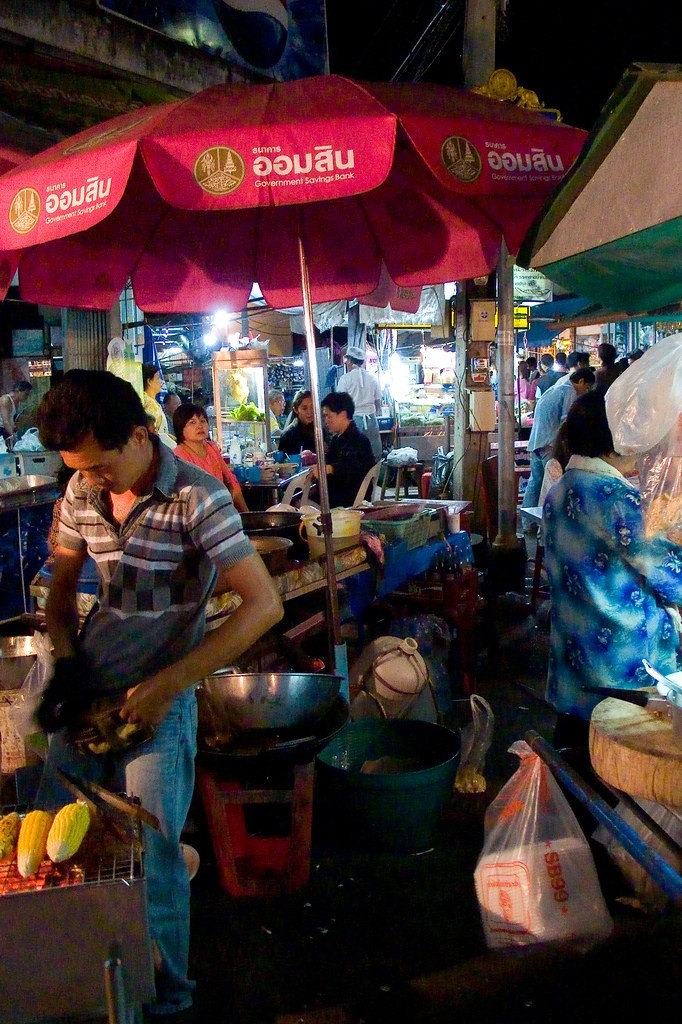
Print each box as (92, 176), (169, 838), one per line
(0, 73), (587, 723)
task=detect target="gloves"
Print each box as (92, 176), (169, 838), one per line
(35, 656), (91, 732)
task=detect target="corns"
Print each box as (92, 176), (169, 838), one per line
(0, 799), (90, 878)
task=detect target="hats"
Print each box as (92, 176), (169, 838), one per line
(344, 347), (367, 360)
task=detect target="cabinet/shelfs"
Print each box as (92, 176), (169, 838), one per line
(214, 349), (271, 457)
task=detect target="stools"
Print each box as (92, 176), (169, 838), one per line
(201, 759), (321, 898)
(515, 467), (533, 502)
(381, 461), (426, 501)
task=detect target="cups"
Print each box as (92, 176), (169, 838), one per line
(233, 451), (307, 484)
(448, 513), (460, 535)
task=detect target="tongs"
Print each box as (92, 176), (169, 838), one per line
(54, 773), (163, 845)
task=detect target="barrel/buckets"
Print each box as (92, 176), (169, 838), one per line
(0, 685), (44, 775)
(298, 510), (363, 562)
(307, 717), (462, 876)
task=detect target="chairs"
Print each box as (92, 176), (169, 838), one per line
(282, 468), (317, 512)
(352, 457), (388, 507)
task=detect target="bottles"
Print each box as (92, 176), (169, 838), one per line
(416, 550), (462, 580)
(348, 634), (427, 723)
(229, 434), (240, 462)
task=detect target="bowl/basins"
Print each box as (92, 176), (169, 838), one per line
(666, 688), (682, 741)
(0, 635), (54, 689)
(248, 537), (291, 571)
(202, 672), (345, 730)
(238, 511), (307, 550)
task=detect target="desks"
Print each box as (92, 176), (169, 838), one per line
(229, 460), (321, 511)
(520, 504), (543, 610)
(448, 440), (530, 452)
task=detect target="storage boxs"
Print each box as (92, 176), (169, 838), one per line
(365, 509), (438, 551)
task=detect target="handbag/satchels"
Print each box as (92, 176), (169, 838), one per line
(65, 691), (157, 766)
(454, 694), (494, 793)
(475, 742), (612, 960)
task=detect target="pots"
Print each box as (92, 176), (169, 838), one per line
(193, 688), (349, 775)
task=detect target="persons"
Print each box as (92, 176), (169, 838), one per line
(37, 363), (285, 1016)
(0, 381), (32, 440)
(279, 391), (327, 455)
(519, 342), (644, 493)
(522, 367), (595, 535)
(542, 387), (682, 918)
(310, 392), (376, 508)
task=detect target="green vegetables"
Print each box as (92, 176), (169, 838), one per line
(229, 402), (264, 422)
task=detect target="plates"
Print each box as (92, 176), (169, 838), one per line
(657, 671), (682, 697)
